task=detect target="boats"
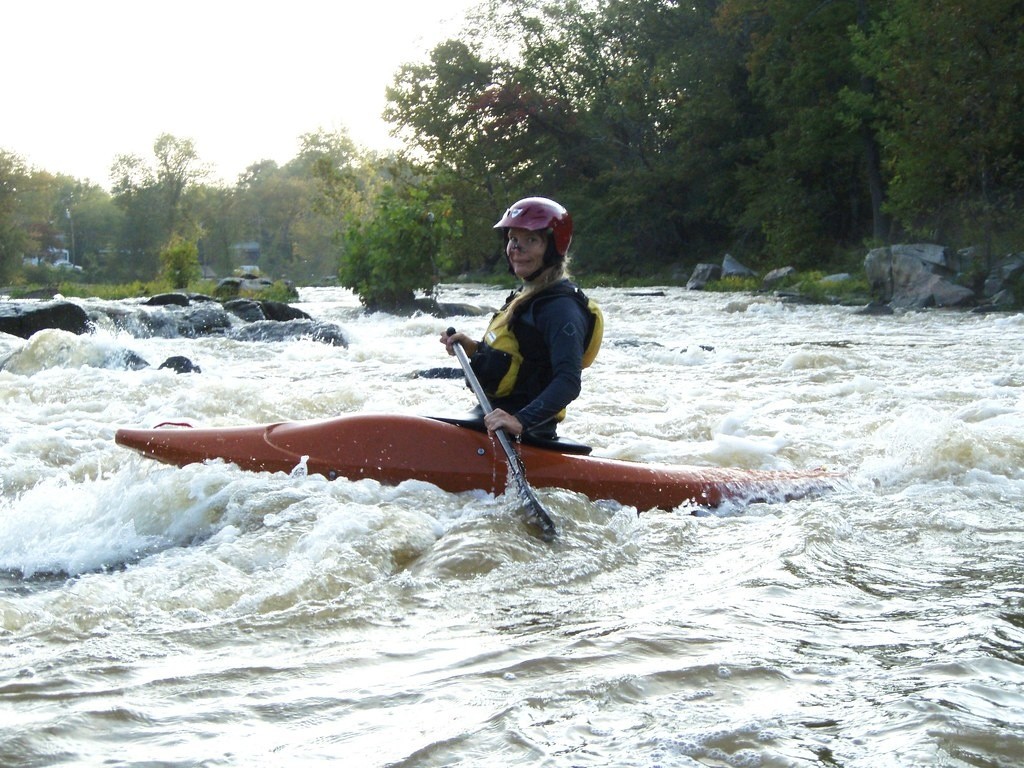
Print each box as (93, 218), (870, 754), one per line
(113, 412), (853, 513)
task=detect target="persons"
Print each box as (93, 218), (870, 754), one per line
(440, 197), (589, 440)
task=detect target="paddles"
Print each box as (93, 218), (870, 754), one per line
(445, 327), (553, 535)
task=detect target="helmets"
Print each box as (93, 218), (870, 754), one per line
(493, 196), (573, 255)
